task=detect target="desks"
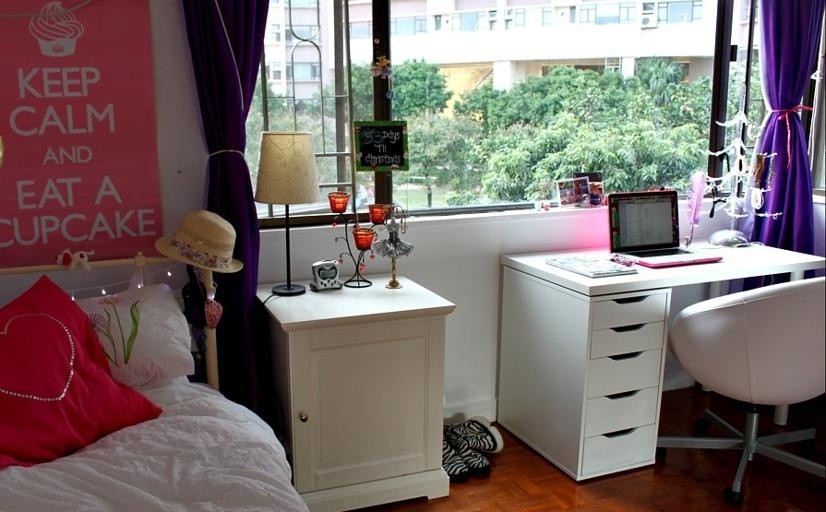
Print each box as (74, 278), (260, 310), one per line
(494, 238), (825, 485)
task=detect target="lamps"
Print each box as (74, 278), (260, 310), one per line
(328, 191), (394, 288)
(254, 132), (321, 299)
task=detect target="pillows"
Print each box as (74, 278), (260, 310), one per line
(0, 275), (196, 471)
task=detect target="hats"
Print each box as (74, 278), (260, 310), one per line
(154, 210), (244, 273)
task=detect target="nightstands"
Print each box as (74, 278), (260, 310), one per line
(258, 272), (457, 511)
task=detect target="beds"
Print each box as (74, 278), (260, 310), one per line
(0, 255), (296, 510)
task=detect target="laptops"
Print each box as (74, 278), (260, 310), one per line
(606, 190), (723, 268)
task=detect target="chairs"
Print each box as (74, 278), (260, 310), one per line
(656, 276), (826, 504)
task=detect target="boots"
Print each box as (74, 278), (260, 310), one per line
(443, 415), (504, 484)
